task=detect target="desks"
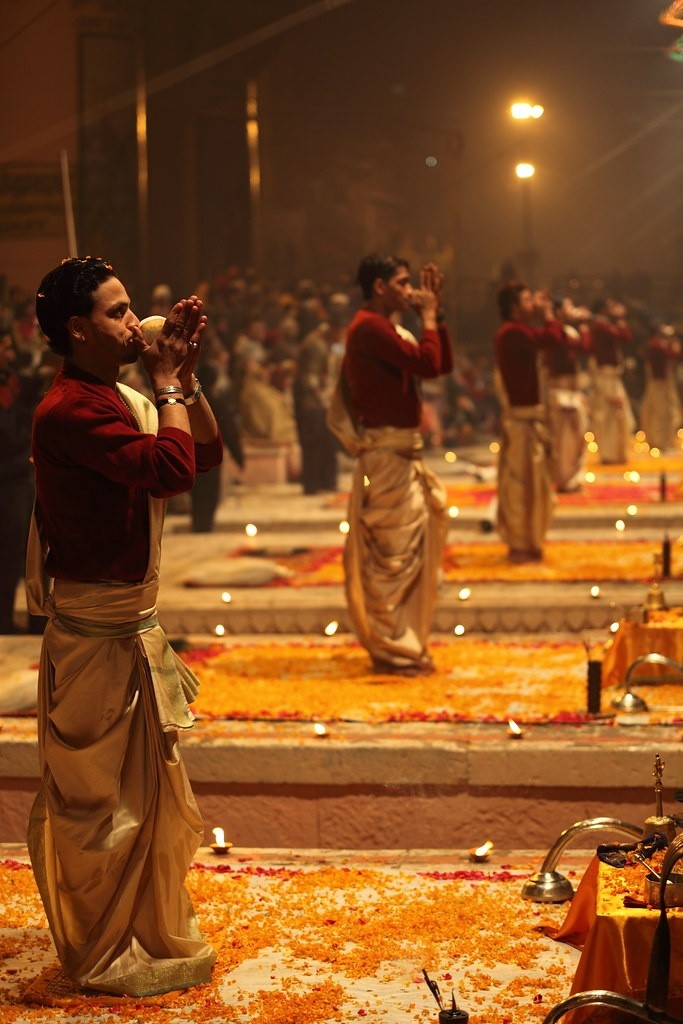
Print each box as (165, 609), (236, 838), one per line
(521, 603), (683, 1023)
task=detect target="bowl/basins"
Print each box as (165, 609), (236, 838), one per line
(644, 871), (683, 907)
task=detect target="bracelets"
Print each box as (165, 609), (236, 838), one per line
(183, 378), (202, 406)
(157, 397), (187, 409)
(436, 314), (446, 323)
(155, 385), (184, 398)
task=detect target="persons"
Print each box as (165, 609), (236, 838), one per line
(294, 299), (338, 494)
(491, 281), (563, 564)
(1, 330), (40, 635)
(541, 293), (596, 494)
(25, 258), (228, 993)
(324, 252), (457, 678)
(153, 264), (483, 440)
(590, 295), (634, 463)
(189, 337), (227, 533)
(641, 320), (682, 453)
(1, 274), (66, 395)
